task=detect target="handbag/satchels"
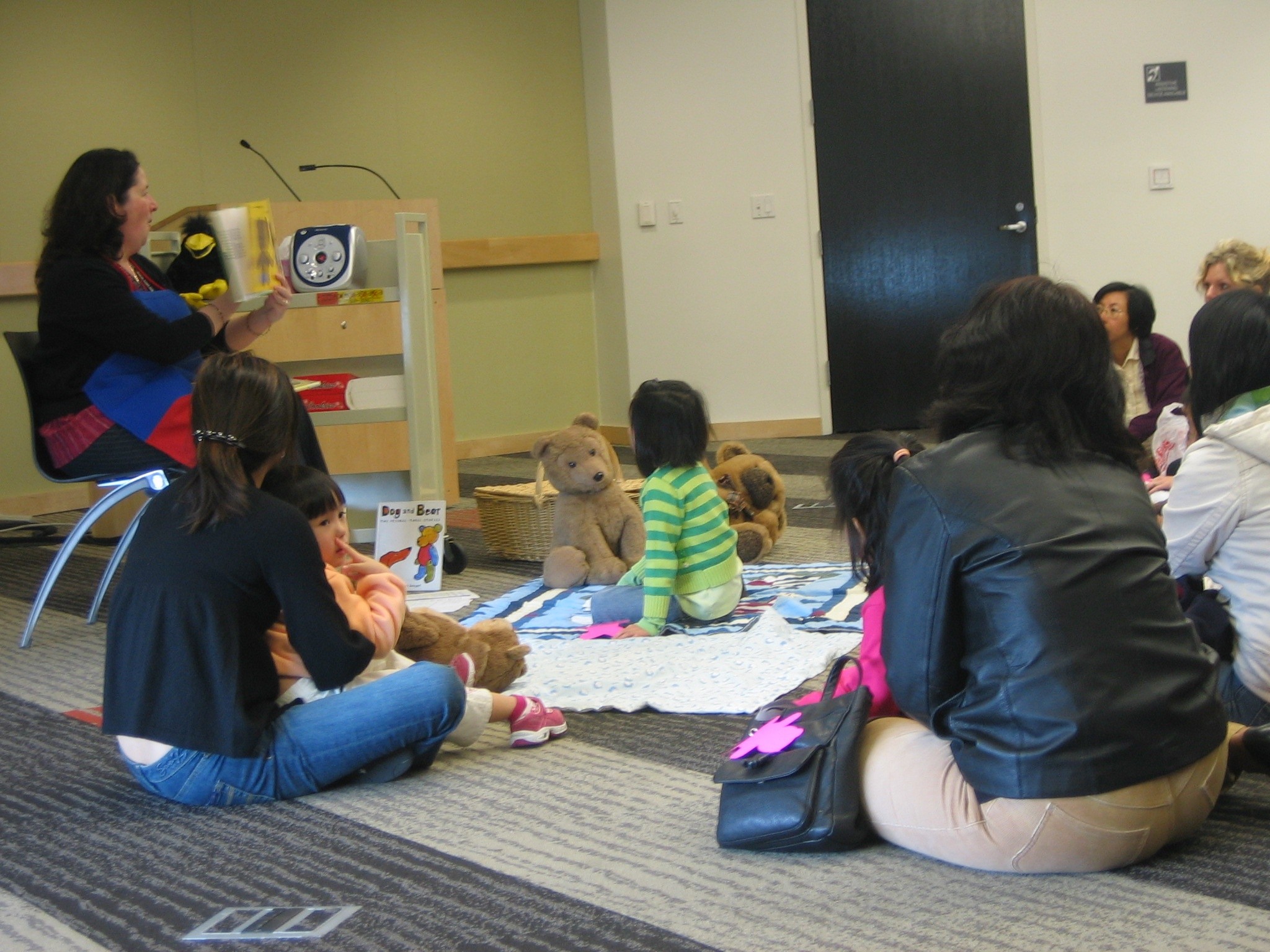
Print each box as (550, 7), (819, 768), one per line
(1152, 402), (1189, 476)
(711, 655), (875, 851)
(1178, 575), (1237, 661)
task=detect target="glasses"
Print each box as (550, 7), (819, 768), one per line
(1199, 281), (1239, 296)
(1096, 305), (1128, 318)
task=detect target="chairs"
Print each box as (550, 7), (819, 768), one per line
(4, 332), (191, 647)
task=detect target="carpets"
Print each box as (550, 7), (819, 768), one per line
(433, 561), (870, 715)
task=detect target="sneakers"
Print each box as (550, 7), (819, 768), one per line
(450, 652), (475, 688)
(511, 695), (568, 747)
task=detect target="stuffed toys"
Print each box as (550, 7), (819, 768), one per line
(392, 607), (531, 694)
(164, 214), (229, 308)
(533, 413), (646, 588)
(710, 441), (788, 565)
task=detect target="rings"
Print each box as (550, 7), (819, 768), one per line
(284, 300), (289, 305)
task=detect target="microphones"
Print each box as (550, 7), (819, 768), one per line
(298, 164), (400, 200)
(240, 140), (301, 201)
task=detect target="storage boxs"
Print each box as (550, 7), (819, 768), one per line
(294, 373), (357, 413)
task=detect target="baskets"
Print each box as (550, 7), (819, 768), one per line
(473, 460), (646, 562)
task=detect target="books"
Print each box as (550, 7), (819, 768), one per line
(374, 500), (447, 591)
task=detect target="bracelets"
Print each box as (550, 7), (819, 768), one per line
(246, 310), (271, 336)
(208, 302), (226, 327)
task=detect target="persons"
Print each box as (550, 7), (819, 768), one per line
(101, 353), (466, 806)
(791, 432), (926, 720)
(1161, 290), (1270, 770)
(1092, 282), (1194, 479)
(266, 463), (568, 747)
(1195, 239), (1270, 303)
(589, 379), (746, 638)
(34, 149), (328, 481)
(855, 276), (1229, 872)
